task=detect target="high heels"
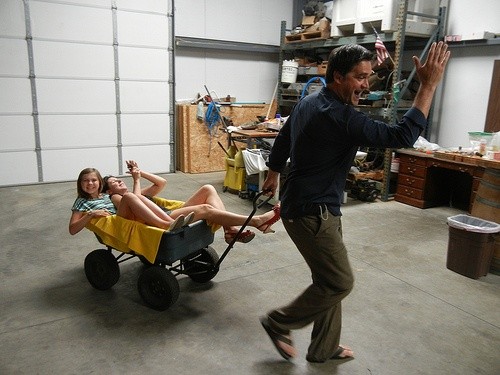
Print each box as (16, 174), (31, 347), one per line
(224, 230), (256, 248)
(257, 201), (281, 235)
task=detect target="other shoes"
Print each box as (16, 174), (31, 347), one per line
(168, 214), (185, 231)
(182, 211), (194, 227)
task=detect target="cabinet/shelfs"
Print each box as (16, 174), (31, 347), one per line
(276, 1), (450, 203)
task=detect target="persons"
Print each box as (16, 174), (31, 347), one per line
(101, 160), (281, 248)
(260, 41), (451, 364)
(69, 168), (195, 235)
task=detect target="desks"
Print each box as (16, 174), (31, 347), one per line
(393, 147), (500, 225)
(218, 124), (280, 150)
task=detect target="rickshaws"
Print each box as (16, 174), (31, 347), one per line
(83, 189), (275, 312)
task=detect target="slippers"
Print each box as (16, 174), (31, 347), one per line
(307, 344), (352, 362)
(260, 312), (295, 362)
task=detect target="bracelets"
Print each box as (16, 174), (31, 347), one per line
(88, 210), (95, 218)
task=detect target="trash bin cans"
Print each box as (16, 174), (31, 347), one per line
(446, 214), (500, 281)
